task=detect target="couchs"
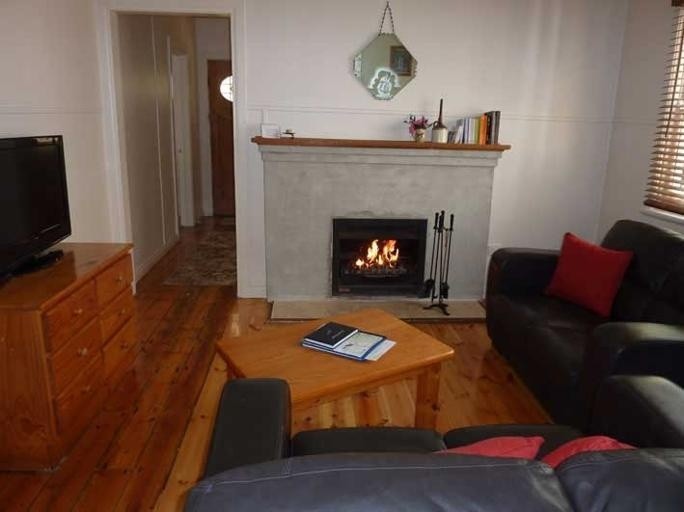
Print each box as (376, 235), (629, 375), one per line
(486, 218), (684, 424)
(185, 373), (684, 512)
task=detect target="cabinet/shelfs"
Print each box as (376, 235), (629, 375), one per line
(0, 242), (140, 469)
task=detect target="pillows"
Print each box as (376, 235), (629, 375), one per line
(543, 435), (639, 468)
(544, 233), (635, 318)
(434, 436), (544, 462)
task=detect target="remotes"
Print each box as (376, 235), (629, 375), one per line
(547, 319), (578, 329)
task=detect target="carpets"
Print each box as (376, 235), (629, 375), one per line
(162, 230), (236, 286)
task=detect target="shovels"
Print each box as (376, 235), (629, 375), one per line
(419, 212), (439, 299)
(441, 214), (454, 300)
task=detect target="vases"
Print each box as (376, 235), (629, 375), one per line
(414, 130), (425, 141)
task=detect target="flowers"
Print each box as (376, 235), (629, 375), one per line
(405, 115), (431, 134)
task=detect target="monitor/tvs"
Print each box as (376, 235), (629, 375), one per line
(0, 135), (72, 278)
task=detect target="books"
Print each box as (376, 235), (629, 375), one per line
(299, 321), (398, 364)
(446, 110), (502, 146)
